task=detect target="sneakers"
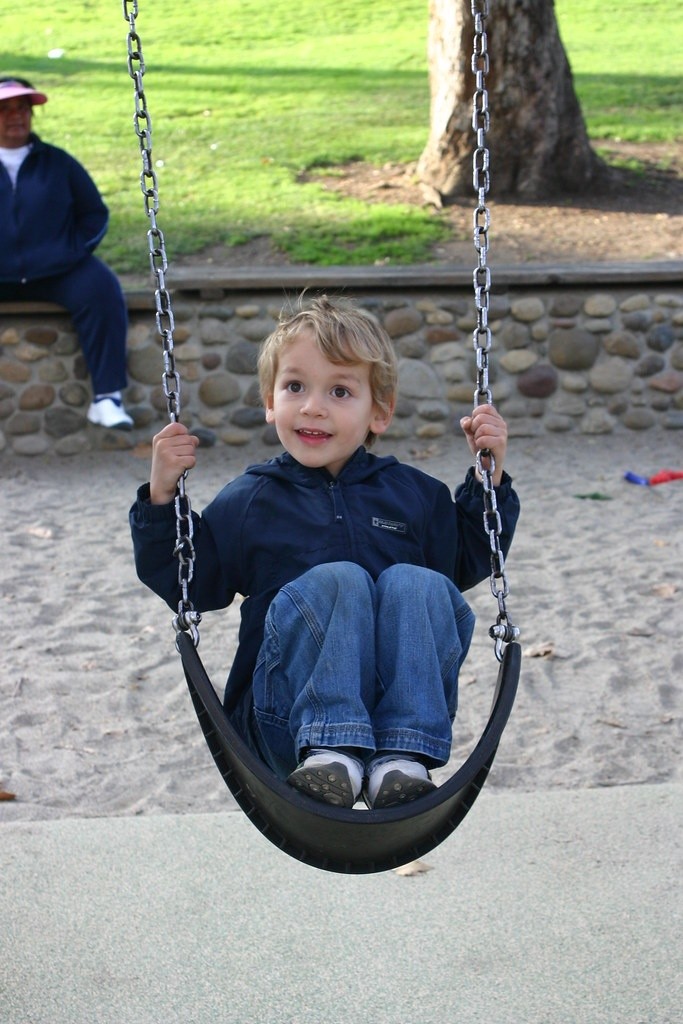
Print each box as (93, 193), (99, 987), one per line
(363, 752), (438, 811)
(87, 390), (134, 431)
(286, 746), (364, 810)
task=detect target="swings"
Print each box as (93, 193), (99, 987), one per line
(122, 0), (522, 876)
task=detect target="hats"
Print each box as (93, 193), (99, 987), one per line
(0, 81), (47, 105)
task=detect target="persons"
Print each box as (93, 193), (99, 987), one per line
(129, 299), (520, 810)
(0, 76), (134, 431)
